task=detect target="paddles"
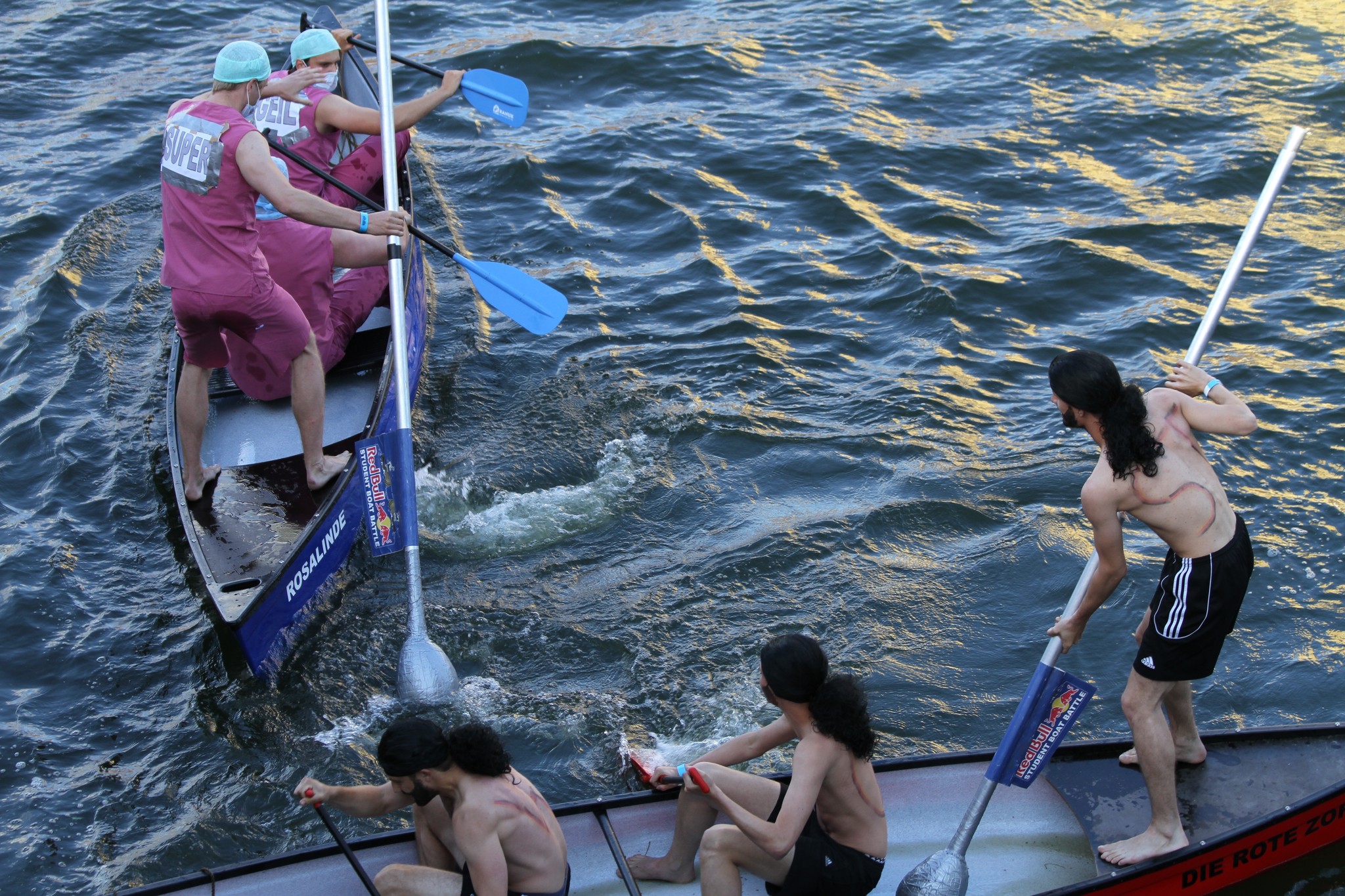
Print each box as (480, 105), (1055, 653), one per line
(306, 788), (380, 896)
(631, 755), (711, 795)
(262, 127), (568, 335)
(299, 10), (529, 129)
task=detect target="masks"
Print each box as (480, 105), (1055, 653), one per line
(302, 60), (339, 92)
(242, 82), (261, 117)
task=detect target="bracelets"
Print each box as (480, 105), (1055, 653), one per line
(1203, 378), (1223, 396)
(675, 763), (687, 776)
(354, 211), (369, 234)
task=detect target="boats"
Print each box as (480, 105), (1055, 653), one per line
(123, 721), (1345, 896)
(165, 4), (426, 675)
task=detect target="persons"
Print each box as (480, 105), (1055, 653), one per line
(1043, 349), (1258, 869)
(158, 30), (467, 501)
(615, 633), (888, 896)
(294, 718), (573, 896)
